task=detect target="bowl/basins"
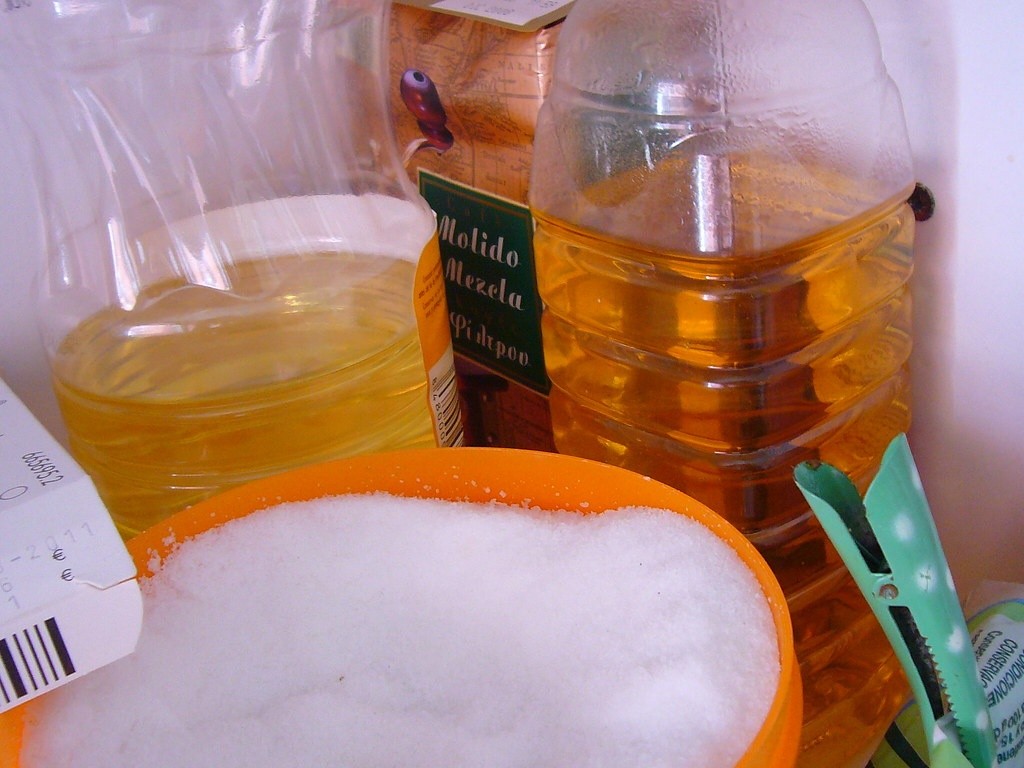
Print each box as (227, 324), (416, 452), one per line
(0, 446), (798, 768)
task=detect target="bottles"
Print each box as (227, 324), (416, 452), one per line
(8, 1), (915, 766)
